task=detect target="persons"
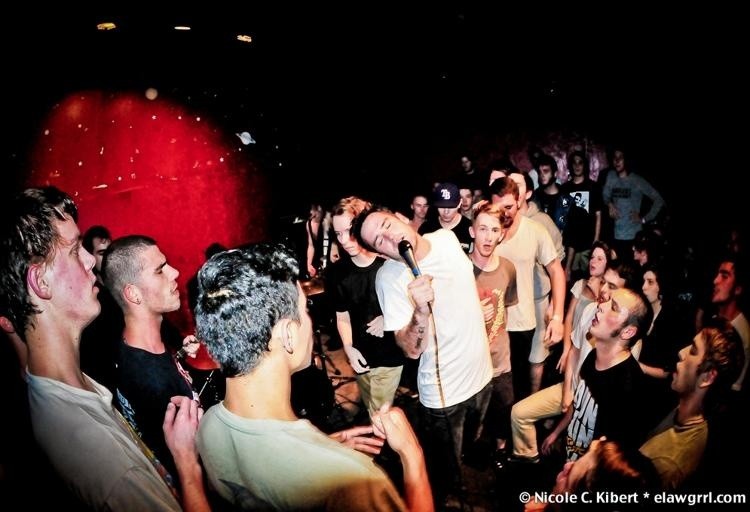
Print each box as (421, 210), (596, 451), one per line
(323, 196), (405, 425)
(305, 154), (470, 279)
(100, 235), (194, 499)
(81, 226), (125, 417)
(186, 243), (228, 328)
(349, 208), (494, 469)
(193, 242), (437, 511)
(0, 185), (209, 512)
(469, 136), (750, 512)
(1, 316), (29, 391)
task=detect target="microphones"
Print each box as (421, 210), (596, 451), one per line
(397, 241), (423, 279)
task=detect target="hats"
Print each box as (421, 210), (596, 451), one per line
(434, 184), (460, 208)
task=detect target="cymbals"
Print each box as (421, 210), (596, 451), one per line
(302, 279), (325, 295)
